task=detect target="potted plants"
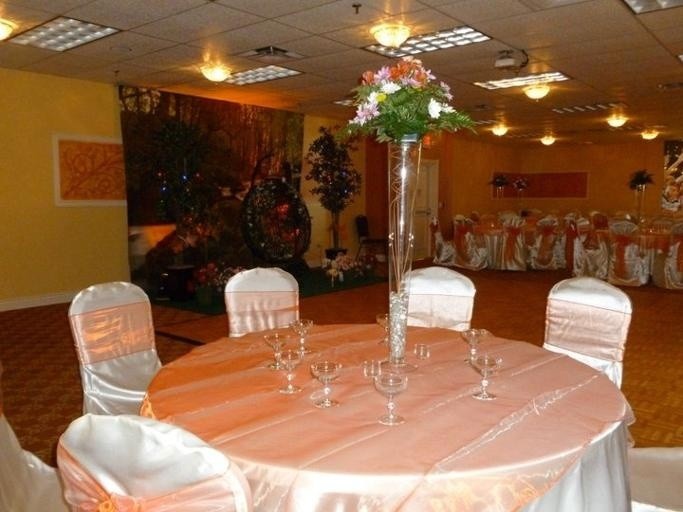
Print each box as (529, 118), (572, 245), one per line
(304, 128), (364, 259)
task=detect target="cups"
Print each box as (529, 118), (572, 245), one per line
(361, 360), (382, 379)
(413, 343), (430, 360)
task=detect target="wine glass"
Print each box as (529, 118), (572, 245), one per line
(264, 334), (289, 369)
(469, 354), (503, 402)
(374, 373), (408, 425)
(288, 319), (313, 352)
(309, 361), (342, 408)
(275, 351), (304, 393)
(460, 329), (489, 366)
(376, 314), (390, 345)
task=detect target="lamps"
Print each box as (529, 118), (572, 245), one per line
(369, 24), (410, 50)
(522, 84), (550, 102)
(200, 64), (231, 84)
(607, 114), (627, 128)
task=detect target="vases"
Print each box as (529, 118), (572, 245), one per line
(635, 185), (646, 220)
(377, 133), (428, 370)
(495, 187), (525, 211)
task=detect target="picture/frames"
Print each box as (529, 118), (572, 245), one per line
(51, 133), (128, 206)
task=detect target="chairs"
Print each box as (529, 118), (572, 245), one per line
(226, 269), (299, 338)
(1, 410), (252, 512)
(401, 267), (475, 332)
(354, 215), (388, 267)
(428, 206), (683, 291)
(542, 276), (633, 389)
(69, 282), (162, 416)
(627, 448), (681, 512)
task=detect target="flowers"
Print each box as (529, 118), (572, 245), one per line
(485, 172), (531, 191)
(328, 54), (483, 149)
(623, 166), (658, 195)
